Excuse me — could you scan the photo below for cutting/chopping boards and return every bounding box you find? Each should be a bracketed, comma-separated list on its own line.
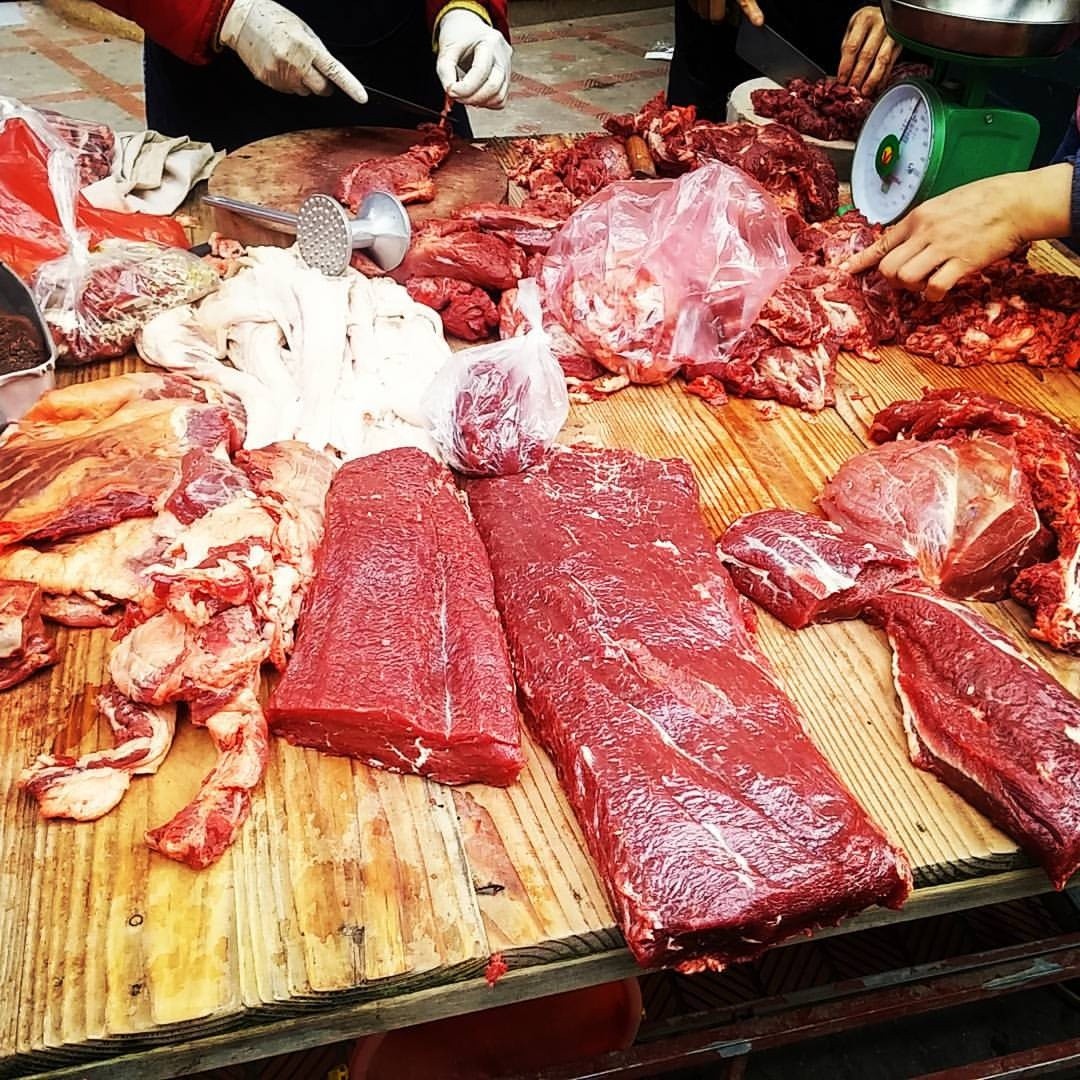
[728, 76, 857, 180]
[206, 128, 509, 248]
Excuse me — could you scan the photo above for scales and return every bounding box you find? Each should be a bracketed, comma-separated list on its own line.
[838, 1, 1079, 229]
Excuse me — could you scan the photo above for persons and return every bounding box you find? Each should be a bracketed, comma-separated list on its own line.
[835, 136, 1080, 303]
[664, 0, 905, 129]
[92, 0, 514, 145]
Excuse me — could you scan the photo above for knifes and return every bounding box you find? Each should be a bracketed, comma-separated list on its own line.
[724, 0, 825, 92]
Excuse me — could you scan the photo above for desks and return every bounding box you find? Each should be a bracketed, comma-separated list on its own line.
[0, 128, 1079, 1080]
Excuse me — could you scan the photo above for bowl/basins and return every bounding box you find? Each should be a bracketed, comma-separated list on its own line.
[0, 261, 60, 427]
